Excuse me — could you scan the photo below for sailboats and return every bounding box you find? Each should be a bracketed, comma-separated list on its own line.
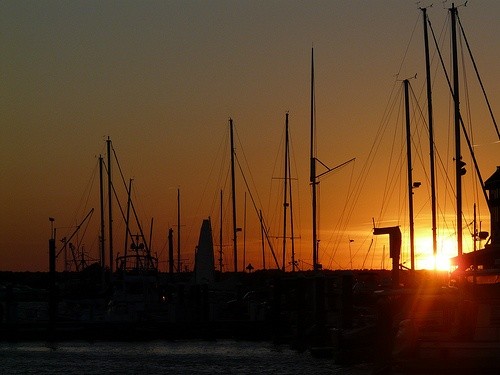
[8, 1, 497, 364]
